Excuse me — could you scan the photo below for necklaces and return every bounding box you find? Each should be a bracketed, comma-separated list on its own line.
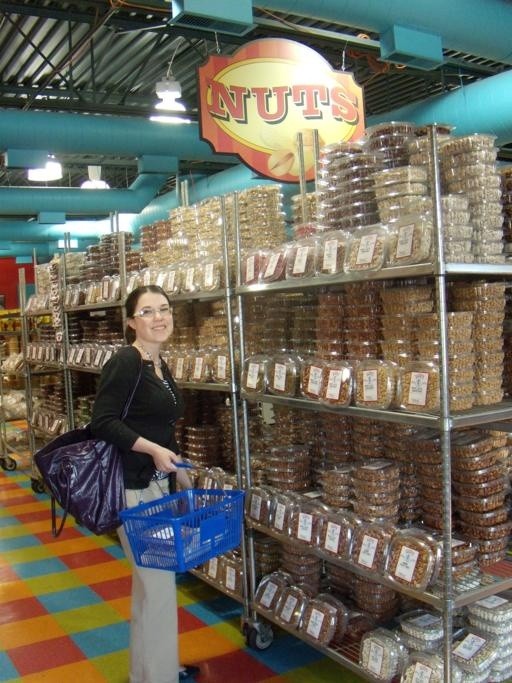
[134, 341, 163, 368]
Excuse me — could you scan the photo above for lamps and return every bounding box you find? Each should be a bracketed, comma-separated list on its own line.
[79, 177, 112, 189]
[148, 37, 191, 126]
[26, 156, 64, 183]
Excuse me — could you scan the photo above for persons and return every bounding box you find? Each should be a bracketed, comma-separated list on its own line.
[90, 281, 203, 682]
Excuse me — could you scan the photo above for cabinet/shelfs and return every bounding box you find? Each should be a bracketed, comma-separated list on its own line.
[124, 189, 250, 602]
[65, 223, 127, 536]
[2, 252, 63, 483]
[230, 130, 511, 641]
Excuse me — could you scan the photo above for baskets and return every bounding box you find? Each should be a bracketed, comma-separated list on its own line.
[117, 462, 245, 573]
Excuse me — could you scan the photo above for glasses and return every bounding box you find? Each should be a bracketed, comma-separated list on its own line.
[130, 306, 173, 320]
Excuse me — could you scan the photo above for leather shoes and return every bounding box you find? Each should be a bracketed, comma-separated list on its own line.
[178, 664, 201, 683]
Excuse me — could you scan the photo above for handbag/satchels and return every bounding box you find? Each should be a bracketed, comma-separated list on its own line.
[32, 424, 130, 539]
